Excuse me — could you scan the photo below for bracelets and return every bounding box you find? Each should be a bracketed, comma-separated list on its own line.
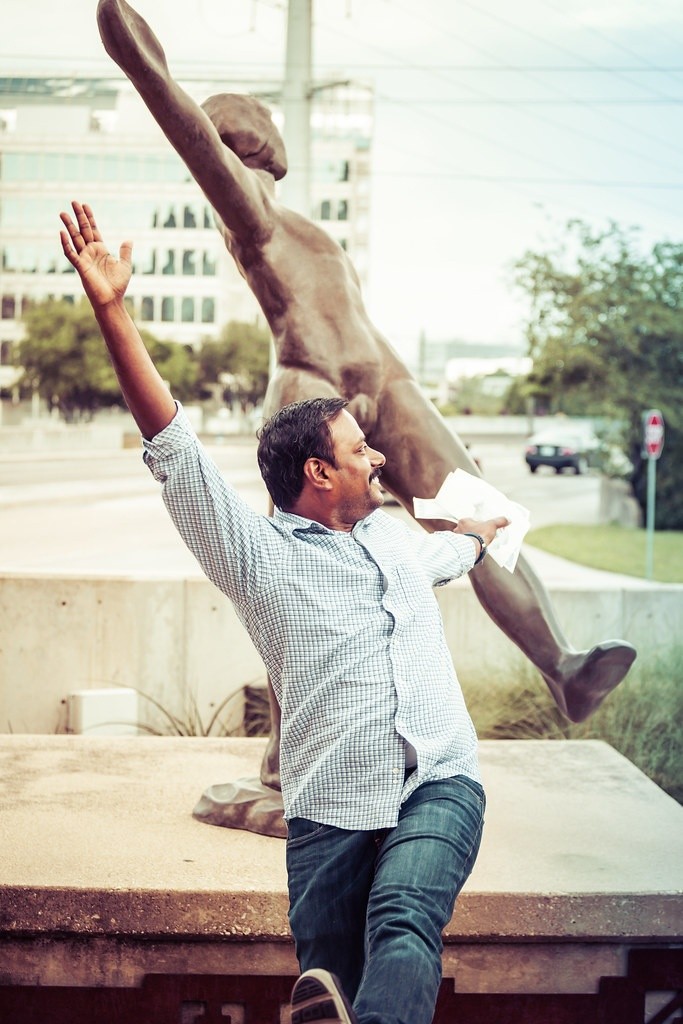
[464, 532, 486, 565]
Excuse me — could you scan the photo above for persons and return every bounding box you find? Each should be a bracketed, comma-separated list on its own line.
[96, 0, 638, 788]
[59, 201, 507, 1024]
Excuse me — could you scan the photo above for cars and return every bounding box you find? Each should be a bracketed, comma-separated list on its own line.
[525, 429, 610, 475]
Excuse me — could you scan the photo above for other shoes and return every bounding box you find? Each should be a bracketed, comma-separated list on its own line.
[288, 966, 361, 1024]
